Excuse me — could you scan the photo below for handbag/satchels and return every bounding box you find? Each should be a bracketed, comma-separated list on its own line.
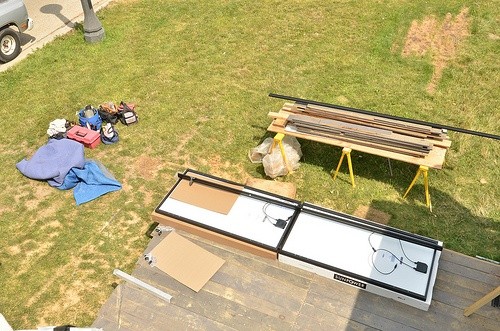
[98, 100, 138, 145]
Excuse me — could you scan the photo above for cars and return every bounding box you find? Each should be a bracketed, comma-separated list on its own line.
[0, 0, 33, 63]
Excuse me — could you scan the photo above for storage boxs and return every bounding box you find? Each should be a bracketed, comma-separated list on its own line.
[67, 124, 101, 149]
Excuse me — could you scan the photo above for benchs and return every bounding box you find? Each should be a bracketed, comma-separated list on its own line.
[333, 148, 355, 186]
[402, 165, 431, 209]
[266, 133, 290, 175]
